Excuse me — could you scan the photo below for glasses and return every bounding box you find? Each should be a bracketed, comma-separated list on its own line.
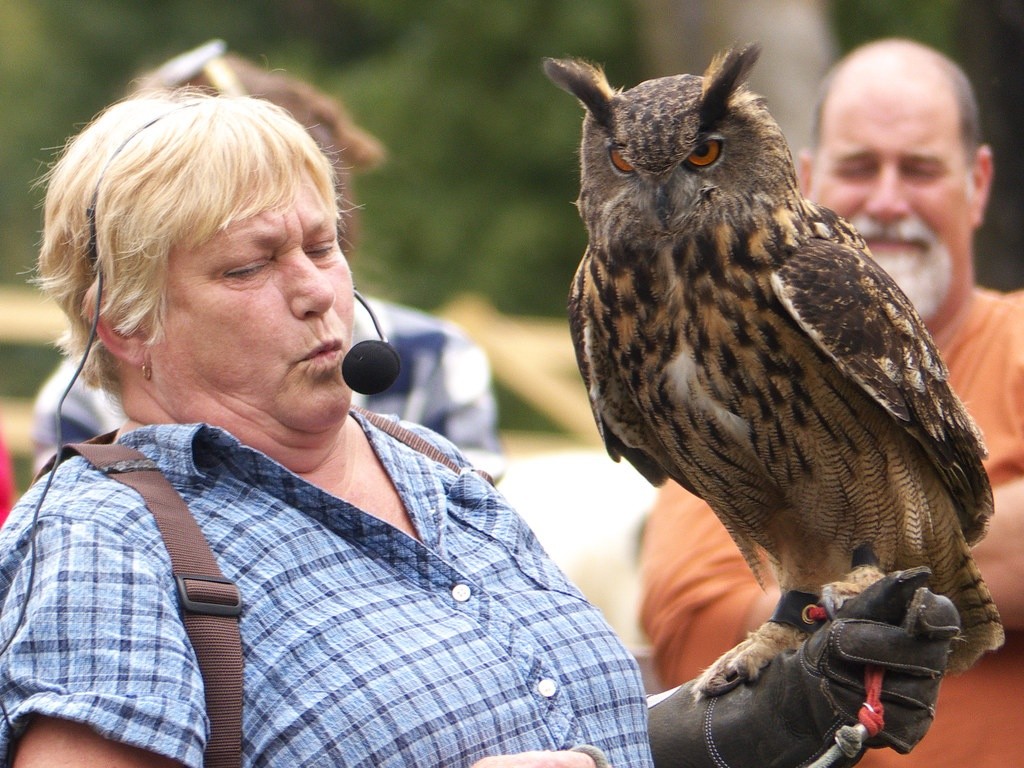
[154, 37, 246, 98]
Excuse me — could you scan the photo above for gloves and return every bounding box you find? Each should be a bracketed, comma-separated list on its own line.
[646, 543, 962, 768]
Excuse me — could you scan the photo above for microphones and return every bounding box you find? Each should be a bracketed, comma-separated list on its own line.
[342, 290, 401, 395]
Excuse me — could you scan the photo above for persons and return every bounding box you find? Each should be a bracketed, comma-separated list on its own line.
[634, 36, 1024, 768]
[29, 41, 504, 484]
[0, 88, 961, 768]
